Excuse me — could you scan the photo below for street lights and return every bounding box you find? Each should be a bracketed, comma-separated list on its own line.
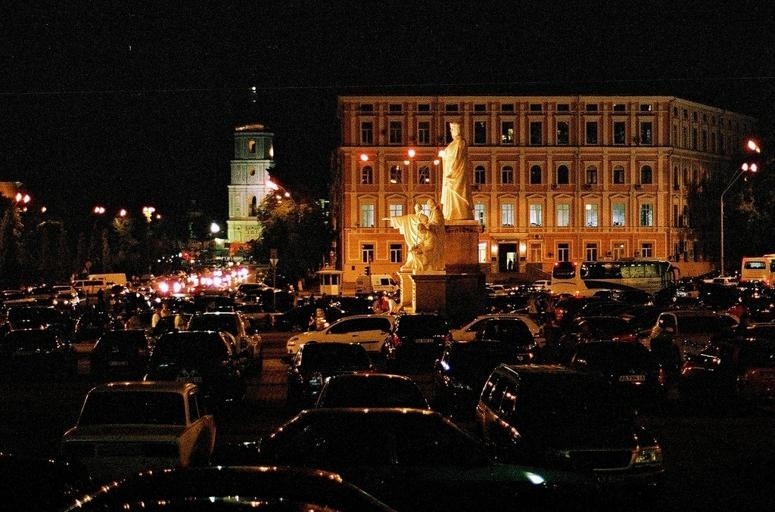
[360, 147, 441, 310]
[0, 192, 221, 254]
[721, 139, 762, 274]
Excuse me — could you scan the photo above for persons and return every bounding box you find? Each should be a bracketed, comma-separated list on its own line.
[433, 122, 475, 221]
[379, 198, 444, 272]
